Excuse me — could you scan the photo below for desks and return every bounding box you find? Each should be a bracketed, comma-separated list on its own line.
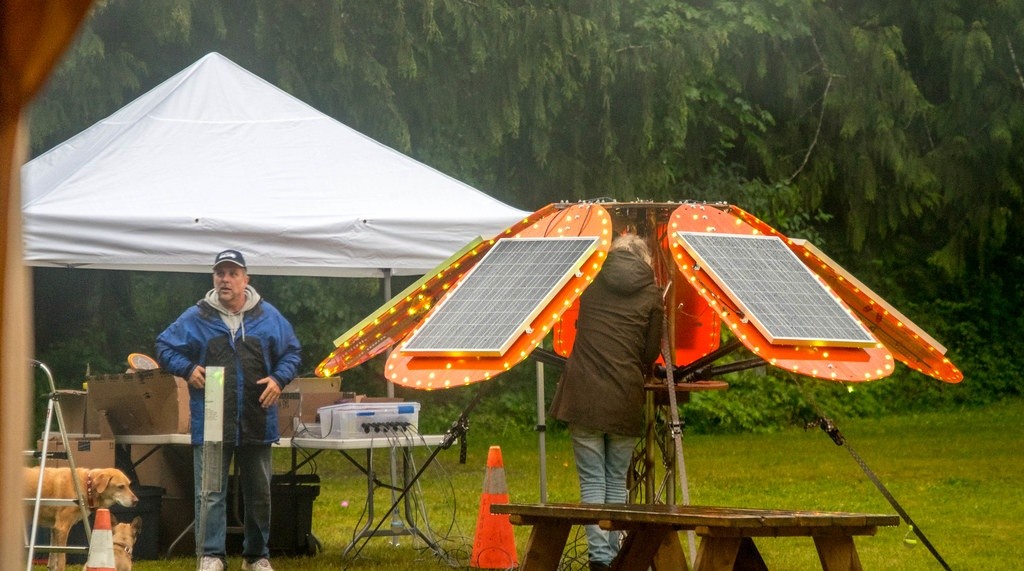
[490, 502, 900, 571]
[102, 432, 458, 561]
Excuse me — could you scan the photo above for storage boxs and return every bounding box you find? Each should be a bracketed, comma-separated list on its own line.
[37, 439, 115, 468]
[275, 376, 344, 437]
[56, 390, 114, 439]
[136, 368, 191, 434]
[317, 402, 421, 440]
[86, 373, 156, 434]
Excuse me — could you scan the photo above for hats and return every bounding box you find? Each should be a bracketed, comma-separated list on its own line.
[212, 250, 245, 270]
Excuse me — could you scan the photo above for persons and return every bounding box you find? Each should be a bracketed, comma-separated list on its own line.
[153, 250, 301, 570]
[553, 232, 665, 571]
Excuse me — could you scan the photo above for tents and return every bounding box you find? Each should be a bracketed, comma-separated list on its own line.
[19, 52, 546, 530]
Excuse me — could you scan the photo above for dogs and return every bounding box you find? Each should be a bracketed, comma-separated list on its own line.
[20, 466, 143, 570]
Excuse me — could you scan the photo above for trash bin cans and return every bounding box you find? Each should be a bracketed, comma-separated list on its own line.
[224, 474, 323, 557]
[109, 485, 166, 560]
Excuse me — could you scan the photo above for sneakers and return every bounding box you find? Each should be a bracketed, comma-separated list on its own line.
[242, 558, 273, 571]
[199, 556, 227, 571]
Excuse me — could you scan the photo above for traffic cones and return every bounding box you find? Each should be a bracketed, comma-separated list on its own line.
[465, 443, 520, 571]
[84, 507, 122, 571]
